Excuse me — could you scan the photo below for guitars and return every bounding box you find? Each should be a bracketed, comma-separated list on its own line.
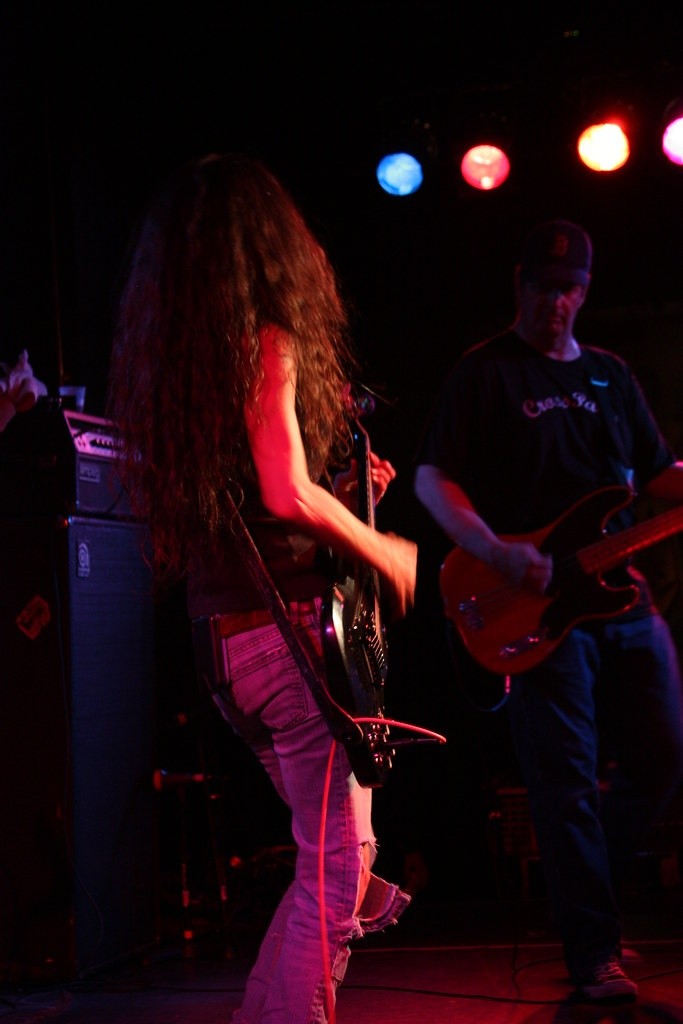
[321, 372, 412, 793]
[435, 480, 683, 676]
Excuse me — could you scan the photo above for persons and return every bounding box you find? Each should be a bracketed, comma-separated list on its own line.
[418, 223, 683, 1000]
[107, 150, 418, 1023]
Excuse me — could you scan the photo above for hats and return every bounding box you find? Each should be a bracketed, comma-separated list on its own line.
[516, 220, 592, 287]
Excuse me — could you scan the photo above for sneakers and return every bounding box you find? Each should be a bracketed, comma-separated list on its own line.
[571, 961, 640, 1004]
